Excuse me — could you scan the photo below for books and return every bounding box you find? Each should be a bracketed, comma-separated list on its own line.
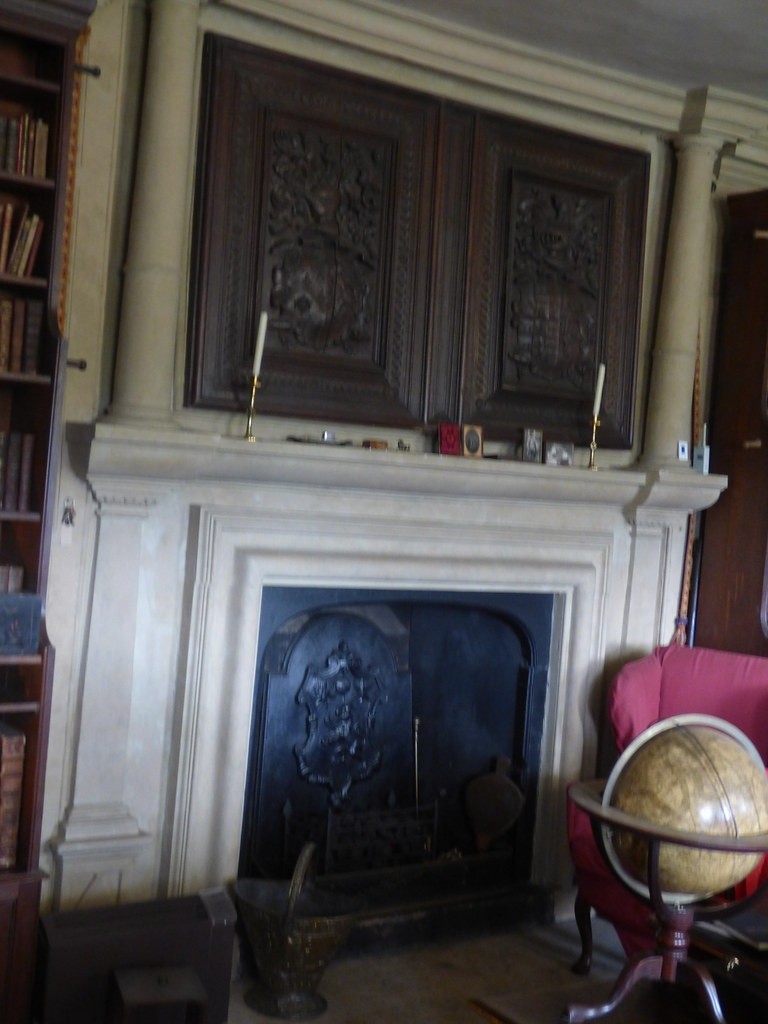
[0, 724, 25, 870]
[0, 107, 50, 593]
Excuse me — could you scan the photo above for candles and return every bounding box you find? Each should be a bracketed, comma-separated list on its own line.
[594, 364, 606, 415]
[252, 310, 269, 376]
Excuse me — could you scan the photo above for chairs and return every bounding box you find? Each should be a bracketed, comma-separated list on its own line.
[561, 645, 768, 1007]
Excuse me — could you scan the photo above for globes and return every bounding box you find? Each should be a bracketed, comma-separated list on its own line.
[562, 714, 768, 1024]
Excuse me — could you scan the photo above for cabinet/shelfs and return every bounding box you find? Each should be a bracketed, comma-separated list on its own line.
[0, 0, 103, 1022]
